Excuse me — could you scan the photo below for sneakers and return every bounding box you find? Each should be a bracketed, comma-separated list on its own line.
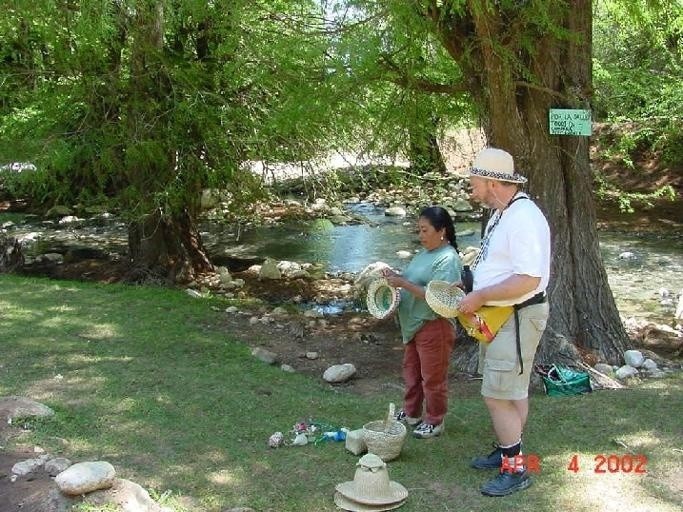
[481, 469, 531, 496]
[471, 442, 502, 468]
[412, 418, 445, 439]
[393, 409, 422, 425]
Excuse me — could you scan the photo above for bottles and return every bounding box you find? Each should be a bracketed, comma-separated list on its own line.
[461, 266, 472, 291]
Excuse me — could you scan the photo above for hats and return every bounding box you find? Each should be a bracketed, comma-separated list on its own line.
[459, 149, 529, 184]
[333, 453, 408, 512]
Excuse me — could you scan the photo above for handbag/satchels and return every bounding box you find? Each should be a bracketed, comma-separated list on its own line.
[543, 365, 592, 397]
[457, 307, 514, 344]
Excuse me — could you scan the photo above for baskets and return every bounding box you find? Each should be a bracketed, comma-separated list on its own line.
[362, 403, 407, 462]
[426, 281, 466, 318]
[366, 279, 401, 319]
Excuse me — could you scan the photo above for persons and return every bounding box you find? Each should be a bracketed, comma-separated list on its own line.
[450, 148, 551, 498]
[384, 207, 462, 439]
